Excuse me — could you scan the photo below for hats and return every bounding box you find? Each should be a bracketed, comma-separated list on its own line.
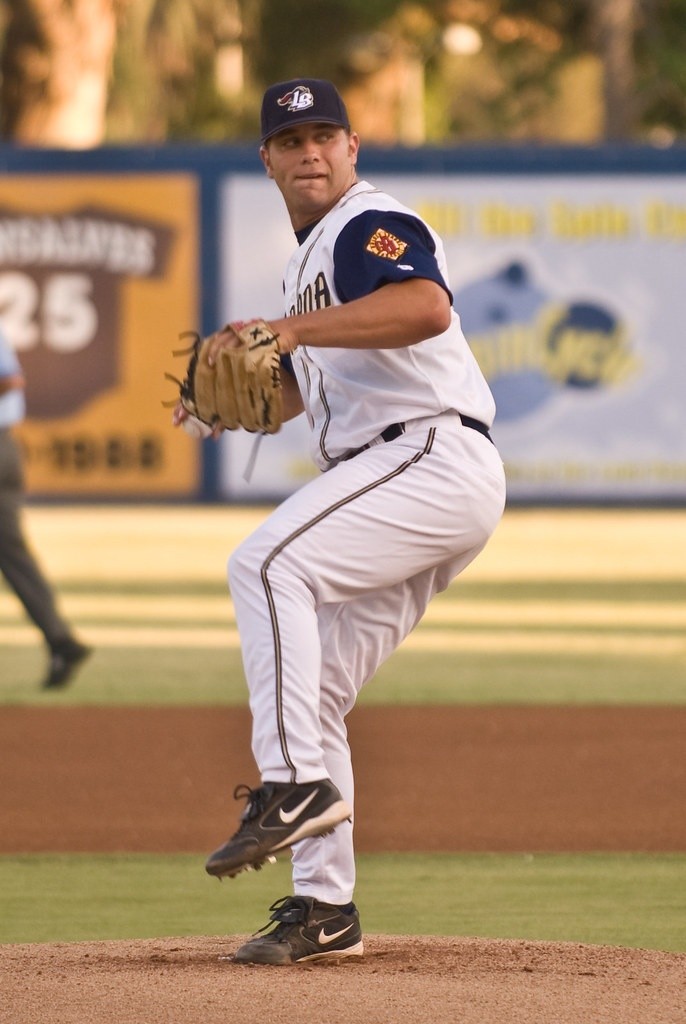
[257, 79, 350, 144]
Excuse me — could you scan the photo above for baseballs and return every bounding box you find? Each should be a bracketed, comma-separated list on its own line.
[182, 414, 213, 439]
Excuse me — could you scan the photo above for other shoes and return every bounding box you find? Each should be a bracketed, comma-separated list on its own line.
[39, 637, 89, 691]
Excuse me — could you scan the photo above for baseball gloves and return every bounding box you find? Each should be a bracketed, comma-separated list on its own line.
[180, 315, 285, 434]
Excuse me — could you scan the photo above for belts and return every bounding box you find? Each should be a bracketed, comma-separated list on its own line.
[352, 411, 491, 459]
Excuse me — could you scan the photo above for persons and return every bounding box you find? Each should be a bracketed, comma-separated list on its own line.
[174, 80, 507, 965]
[0, 331, 93, 689]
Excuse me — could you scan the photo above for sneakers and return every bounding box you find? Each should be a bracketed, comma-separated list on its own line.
[205, 778, 350, 880]
[231, 895, 364, 968]
